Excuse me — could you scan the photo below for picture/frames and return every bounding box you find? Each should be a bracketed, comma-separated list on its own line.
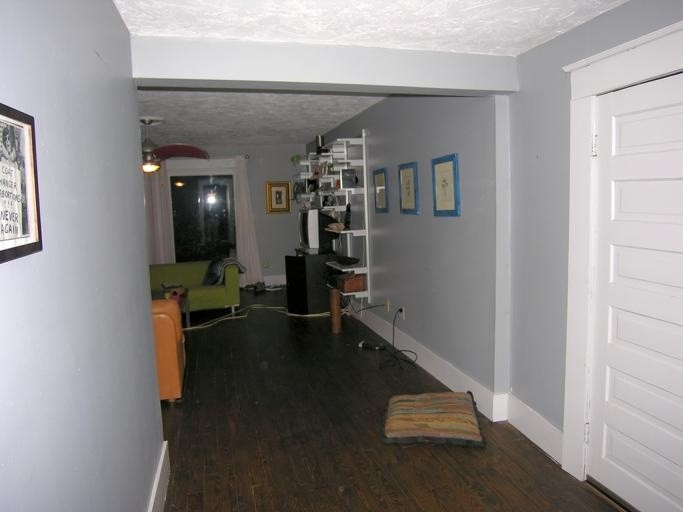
[0, 102, 42, 264]
[264, 179, 292, 216]
[372, 152, 463, 218]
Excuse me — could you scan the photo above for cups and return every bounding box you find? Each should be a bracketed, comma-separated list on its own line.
[315, 135, 324, 148]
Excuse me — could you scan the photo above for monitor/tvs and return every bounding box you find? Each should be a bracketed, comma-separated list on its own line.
[296, 208, 339, 250]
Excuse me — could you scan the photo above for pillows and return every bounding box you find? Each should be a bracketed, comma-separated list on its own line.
[380, 388, 487, 450]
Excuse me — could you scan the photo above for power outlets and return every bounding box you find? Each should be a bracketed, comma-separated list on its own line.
[383, 298, 389, 311]
[398, 305, 405, 320]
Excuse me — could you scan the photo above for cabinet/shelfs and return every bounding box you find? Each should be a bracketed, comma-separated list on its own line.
[299, 128, 370, 303]
[284, 248, 337, 315]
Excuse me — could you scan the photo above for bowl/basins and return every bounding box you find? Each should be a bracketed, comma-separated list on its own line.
[332, 255, 359, 266]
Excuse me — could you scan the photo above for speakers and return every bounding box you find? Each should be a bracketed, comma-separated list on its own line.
[305, 178, 315, 192]
[339, 168, 355, 189]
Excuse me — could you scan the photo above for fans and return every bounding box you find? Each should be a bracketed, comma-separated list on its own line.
[138, 118, 210, 162]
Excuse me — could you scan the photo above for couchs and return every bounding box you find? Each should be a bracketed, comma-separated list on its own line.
[151, 299, 186, 403]
[149, 259, 241, 316]
